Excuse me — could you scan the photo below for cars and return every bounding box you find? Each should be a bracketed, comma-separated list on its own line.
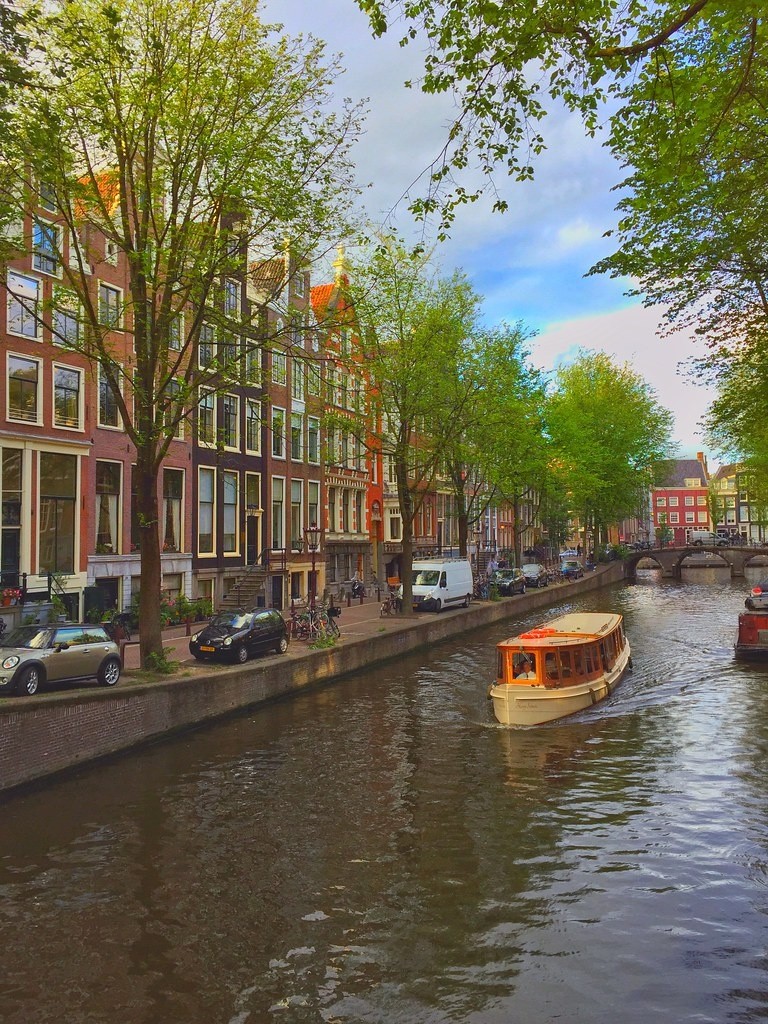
[559, 549, 577, 559]
[520, 563, 549, 588]
[559, 560, 584, 579]
[188, 605, 290, 665]
[0, 621, 122, 696]
[496, 567, 526, 598]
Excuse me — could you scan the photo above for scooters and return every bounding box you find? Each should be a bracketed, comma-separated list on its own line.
[350, 579, 365, 599]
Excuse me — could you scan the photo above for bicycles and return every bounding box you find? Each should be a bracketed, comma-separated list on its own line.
[286, 596, 341, 643]
[380, 591, 399, 616]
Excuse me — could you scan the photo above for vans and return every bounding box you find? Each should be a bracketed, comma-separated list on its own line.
[397, 555, 474, 614]
[690, 531, 730, 547]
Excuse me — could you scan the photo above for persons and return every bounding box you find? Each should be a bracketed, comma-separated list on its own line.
[723, 530, 764, 548]
[543, 644, 599, 679]
[577, 543, 582, 557]
[633, 537, 675, 550]
[513, 659, 536, 680]
[625, 539, 629, 544]
[489, 555, 509, 569]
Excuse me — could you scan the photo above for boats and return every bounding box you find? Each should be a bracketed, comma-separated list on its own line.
[732, 574, 768, 655]
[489, 612, 632, 728]
